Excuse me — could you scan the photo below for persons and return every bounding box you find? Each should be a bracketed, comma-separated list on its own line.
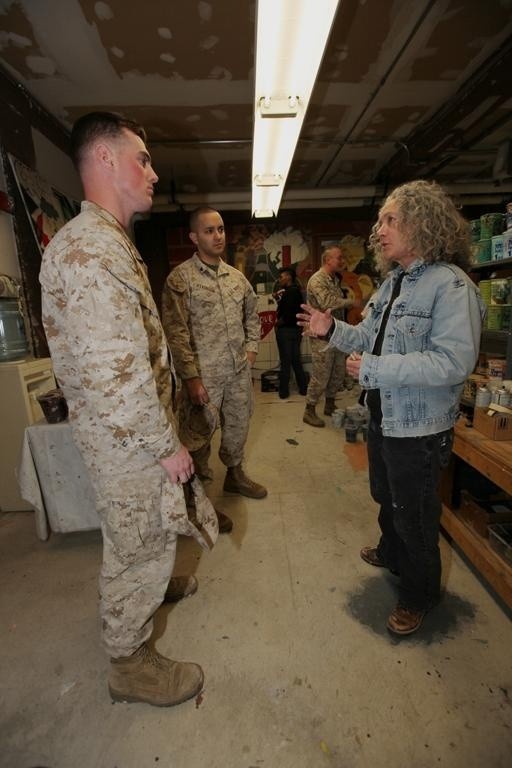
[39, 110, 219, 706]
[302, 246, 355, 427]
[296, 180, 488, 636]
[160, 206, 268, 534]
[272, 267, 307, 399]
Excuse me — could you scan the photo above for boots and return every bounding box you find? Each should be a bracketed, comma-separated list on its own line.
[164, 575, 198, 602]
[108, 642, 204, 707]
[303, 404, 325, 427]
[324, 397, 339, 416]
[223, 464, 267, 499]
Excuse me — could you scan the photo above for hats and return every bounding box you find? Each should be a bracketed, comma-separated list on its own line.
[160, 474, 219, 551]
[179, 401, 218, 453]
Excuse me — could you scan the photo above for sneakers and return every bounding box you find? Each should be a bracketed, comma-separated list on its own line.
[360, 546, 384, 567]
[386, 600, 426, 634]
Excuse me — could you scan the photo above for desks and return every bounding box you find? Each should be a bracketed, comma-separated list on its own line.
[435, 406, 512, 614]
[20, 417, 99, 540]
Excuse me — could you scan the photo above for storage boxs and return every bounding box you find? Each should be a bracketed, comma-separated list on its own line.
[474, 402, 512, 440]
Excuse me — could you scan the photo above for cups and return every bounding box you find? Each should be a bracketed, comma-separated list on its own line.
[331, 405, 370, 444]
[35, 393, 65, 425]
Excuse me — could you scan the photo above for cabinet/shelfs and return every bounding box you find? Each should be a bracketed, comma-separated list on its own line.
[463, 255, 512, 431]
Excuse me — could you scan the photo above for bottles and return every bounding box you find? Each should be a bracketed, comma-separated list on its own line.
[494, 385, 512, 407]
[475, 384, 492, 408]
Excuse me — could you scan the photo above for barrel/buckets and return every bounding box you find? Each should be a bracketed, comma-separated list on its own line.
[0, 296, 29, 362]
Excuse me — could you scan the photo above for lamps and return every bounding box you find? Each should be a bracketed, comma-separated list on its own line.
[251, 0, 341, 218]
